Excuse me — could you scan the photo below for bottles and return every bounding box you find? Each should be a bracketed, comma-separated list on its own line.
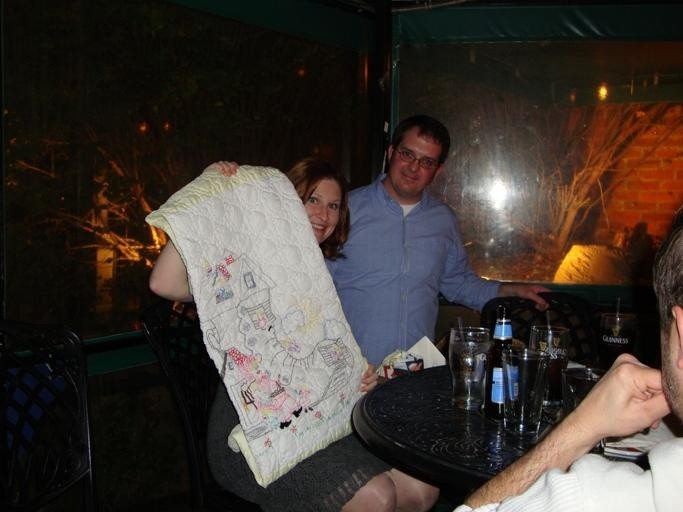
[490, 305, 521, 414]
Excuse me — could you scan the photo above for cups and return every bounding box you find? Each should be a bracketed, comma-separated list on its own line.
[447, 324, 489, 411]
[502, 346, 546, 438]
[600, 313, 637, 360]
[562, 366, 601, 410]
[530, 324, 569, 408]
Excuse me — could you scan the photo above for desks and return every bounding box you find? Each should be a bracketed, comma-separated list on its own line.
[352, 358, 650, 495]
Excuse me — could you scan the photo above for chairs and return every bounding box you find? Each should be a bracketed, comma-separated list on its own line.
[140, 300, 261, 510]
[480, 292, 608, 369]
[0, 317, 96, 512]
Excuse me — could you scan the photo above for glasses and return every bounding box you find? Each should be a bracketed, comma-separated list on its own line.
[395, 148, 436, 169]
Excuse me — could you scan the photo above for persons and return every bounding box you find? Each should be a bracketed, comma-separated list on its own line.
[458, 225, 681, 508]
[319, 115, 553, 376]
[149, 157, 441, 508]
